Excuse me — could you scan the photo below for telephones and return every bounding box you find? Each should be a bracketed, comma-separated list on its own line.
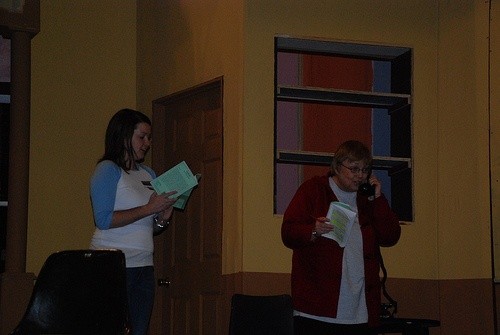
[359, 172, 375, 197]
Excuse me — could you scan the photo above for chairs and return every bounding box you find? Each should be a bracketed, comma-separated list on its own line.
[9, 250, 127, 335]
[229, 294, 293, 335]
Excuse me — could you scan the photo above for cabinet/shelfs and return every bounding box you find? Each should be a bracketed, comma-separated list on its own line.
[274, 37, 413, 224]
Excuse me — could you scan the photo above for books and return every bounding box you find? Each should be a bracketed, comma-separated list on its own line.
[149, 161, 204, 209]
[321, 203, 358, 247]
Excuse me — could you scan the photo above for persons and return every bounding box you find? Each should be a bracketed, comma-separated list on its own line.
[282, 140, 401, 334]
[89, 108, 178, 335]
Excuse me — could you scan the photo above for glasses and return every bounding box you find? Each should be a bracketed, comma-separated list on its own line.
[340, 162, 371, 174]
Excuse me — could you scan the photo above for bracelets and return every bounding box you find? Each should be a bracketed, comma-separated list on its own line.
[155, 218, 170, 228]
